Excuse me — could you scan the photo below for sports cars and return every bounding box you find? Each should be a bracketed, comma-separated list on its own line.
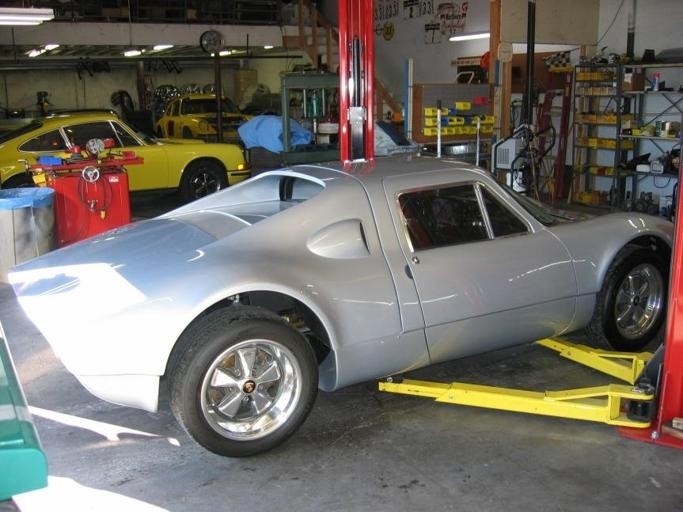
[0, 111, 245, 204]
[7, 154, 673, 457]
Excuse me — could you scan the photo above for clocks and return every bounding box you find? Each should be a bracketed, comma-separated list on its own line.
[496, 42, 512, 63]
[200, 29, 225, 56]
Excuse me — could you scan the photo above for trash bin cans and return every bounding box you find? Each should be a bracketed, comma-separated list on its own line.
[0, 187, 56, 283]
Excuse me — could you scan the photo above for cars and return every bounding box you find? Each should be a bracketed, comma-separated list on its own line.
[154, 96, 254, 146]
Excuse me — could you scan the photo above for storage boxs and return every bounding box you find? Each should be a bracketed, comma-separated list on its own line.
[621, 72, 643, 93]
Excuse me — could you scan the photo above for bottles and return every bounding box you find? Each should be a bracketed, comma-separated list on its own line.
[652, 72, 660, 91]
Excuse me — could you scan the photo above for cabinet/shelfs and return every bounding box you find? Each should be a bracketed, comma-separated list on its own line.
[620, 59, 683, 222]
[568, 59, 626, 209]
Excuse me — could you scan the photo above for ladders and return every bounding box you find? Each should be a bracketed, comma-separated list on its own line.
[534, 65, 574, 204]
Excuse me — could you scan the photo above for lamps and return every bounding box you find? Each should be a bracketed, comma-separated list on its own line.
[0, 0, 55, 27]
[447, 32, 490, 42]
